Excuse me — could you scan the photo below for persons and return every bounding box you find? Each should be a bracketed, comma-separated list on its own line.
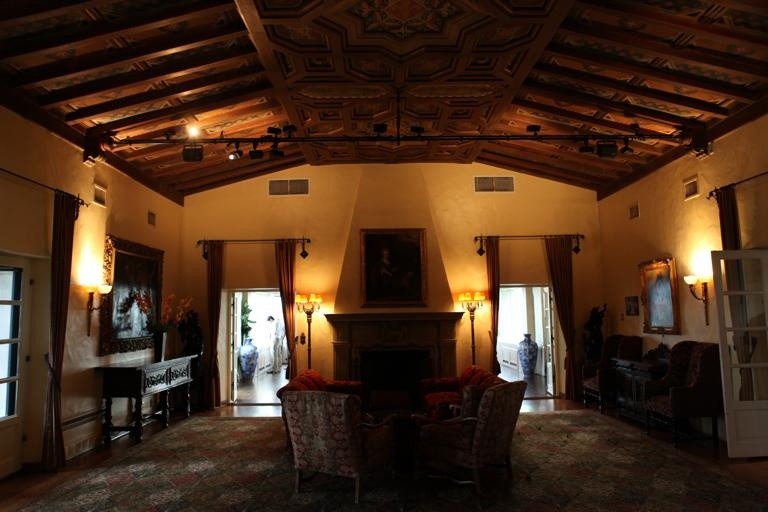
[266, 314, 286, 374]
[378, 248, 394, 278]
[650, 272, 672, 316]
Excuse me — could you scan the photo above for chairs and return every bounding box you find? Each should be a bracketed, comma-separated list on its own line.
[426, 381, 529, 511]
[282, 391, 394, 505]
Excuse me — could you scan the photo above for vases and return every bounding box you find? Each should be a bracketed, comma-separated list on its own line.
[518, 334, 538, 380]
[238, 339, 258, 384]
[152, 331, 167, 362]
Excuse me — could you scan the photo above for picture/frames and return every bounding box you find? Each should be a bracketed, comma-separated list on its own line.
[623, 296, 639, 316]
[637, 257, 679, 335]
[99, 235, 164, 356]
[359, 227, 429, 307]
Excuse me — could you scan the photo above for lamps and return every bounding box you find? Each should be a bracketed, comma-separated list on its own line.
[683, 250, 716, 326]
[294, 293, 323, 370]
[99, 87, 693, 161]
[459, 292, 483, 366]
[87, 237, 115, 336]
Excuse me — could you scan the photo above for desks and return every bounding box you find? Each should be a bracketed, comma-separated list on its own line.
[612, 342, 671, 423]
[93, 353, 199, 444]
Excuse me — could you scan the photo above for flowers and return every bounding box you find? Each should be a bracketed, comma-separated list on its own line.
[135, 290, 194, 331]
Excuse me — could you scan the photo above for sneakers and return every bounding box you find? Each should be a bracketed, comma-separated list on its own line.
[267, 371, 276, 374]
[277, 369, 280, 373]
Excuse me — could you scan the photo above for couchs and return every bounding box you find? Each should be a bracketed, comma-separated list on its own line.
[579, 334, 643, 415]
[639, 341, 723, 444]
[411, 367, 508, 463]
[277, 370, 363, 447]
